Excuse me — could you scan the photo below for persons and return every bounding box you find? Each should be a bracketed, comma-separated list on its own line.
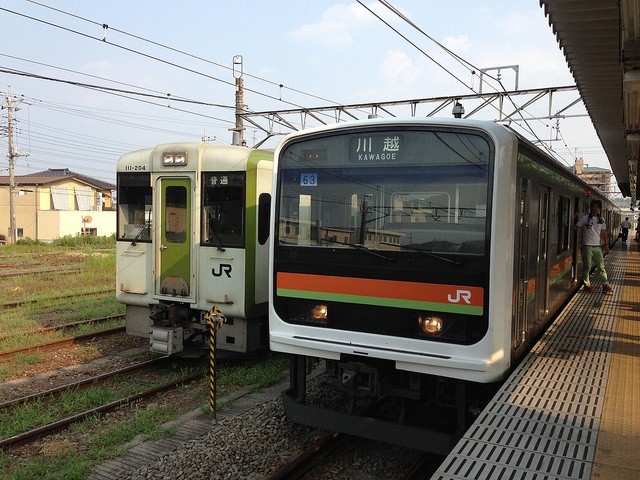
[621, 217, 631, 241]
[634, 219, 640, 240]
[575, 200, 614, 293]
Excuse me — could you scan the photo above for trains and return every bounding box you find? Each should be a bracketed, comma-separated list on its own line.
[269, 116, 622, 457]
[115, 142, 274, 356]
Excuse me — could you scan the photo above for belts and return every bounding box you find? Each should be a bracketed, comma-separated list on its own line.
[584, 245, 600, 247]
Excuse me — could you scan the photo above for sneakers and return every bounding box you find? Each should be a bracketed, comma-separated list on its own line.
[585, 286, 595, 291]
[603, 285, 614, 292]
